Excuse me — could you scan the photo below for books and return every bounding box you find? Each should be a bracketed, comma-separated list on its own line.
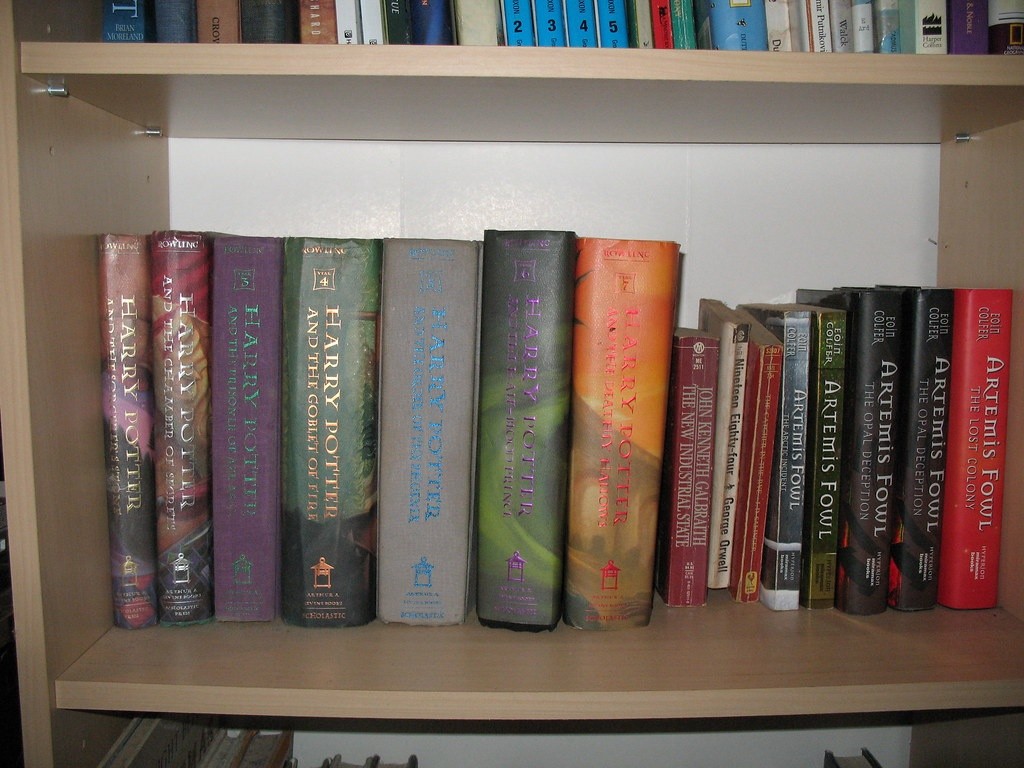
[101, 0, 1024, 54]
[824, 747, 882, 768]
[96, 229, 1014, 640]
[96, 718, 418, 768]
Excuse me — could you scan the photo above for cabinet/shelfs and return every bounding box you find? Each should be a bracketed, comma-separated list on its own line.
[0, 0, 1024, 768]
